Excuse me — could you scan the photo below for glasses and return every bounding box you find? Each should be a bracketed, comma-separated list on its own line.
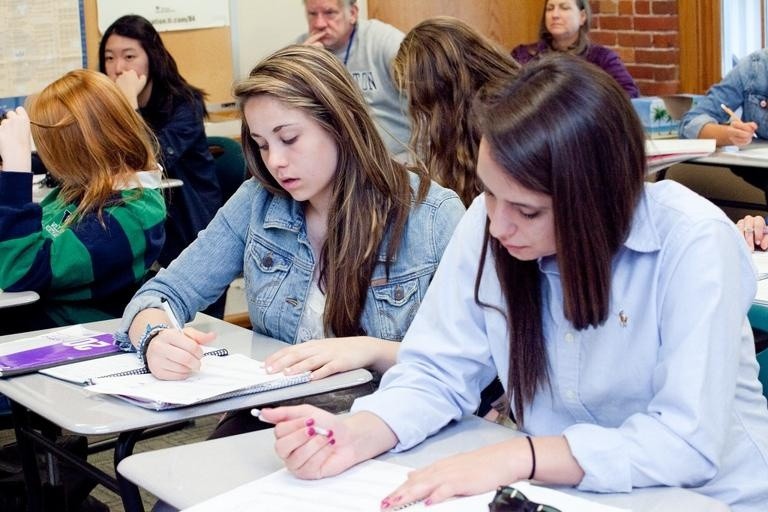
[488, 485, 560, 511]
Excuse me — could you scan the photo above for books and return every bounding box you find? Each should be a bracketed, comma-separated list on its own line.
[42, 343, 312, 413]
[643, 137, 719, 175]
[178, 456, 499, 512]
[720, 147, 767, 160]
[0, 332, 123, 378]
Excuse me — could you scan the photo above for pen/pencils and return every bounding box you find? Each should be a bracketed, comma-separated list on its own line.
[161, 296, 187, 338]
[249, 407, 331, 439]
[720, 103, 759, 140]
[742, 226, 768, 235]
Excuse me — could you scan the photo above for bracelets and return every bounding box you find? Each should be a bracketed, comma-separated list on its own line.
[135, 323, 170, 374]
[524, 435, 538, 479]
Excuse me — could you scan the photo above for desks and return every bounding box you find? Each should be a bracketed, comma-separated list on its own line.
[660, 132, 768, 217]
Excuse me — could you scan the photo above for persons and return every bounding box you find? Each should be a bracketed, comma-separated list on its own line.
[294, 0, 415, 170]
[735, 212, 768, 253]
[97, 13, 225, 276]
[675, 47, 768, 149]
[256, 51, 768, 512]
[506, 1, 641, 99]
[109, 44, 470, 512]
[0, 67, 169, 475]
